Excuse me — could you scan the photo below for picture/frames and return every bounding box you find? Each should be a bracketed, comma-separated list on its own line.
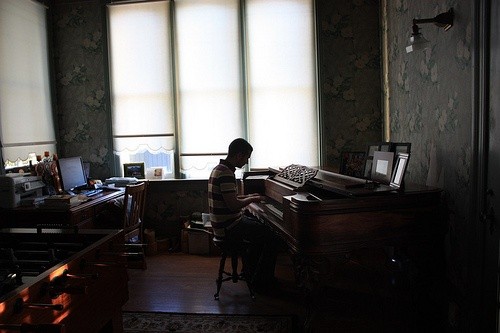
[365, 142, 411, 189]
[124, 163, 145, 179]
[338, 151, 365, 176]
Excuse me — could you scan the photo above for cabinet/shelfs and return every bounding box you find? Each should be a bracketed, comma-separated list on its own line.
[0, 184, 124, 229]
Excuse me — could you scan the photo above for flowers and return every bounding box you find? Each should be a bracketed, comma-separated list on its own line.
[35, 152, 60, 186]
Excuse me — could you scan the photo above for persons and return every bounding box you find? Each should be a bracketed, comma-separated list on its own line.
[207, 138, 279, 295]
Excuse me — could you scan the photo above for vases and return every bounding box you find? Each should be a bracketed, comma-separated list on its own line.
[48, 183, 60, 196]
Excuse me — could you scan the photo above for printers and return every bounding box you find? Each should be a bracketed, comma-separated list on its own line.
[0, 173, 46, 209]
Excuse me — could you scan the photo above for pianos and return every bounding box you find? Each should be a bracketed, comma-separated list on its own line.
[243, 166, 443, 292]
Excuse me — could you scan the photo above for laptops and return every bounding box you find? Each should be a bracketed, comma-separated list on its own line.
[56, 156, 103, 196]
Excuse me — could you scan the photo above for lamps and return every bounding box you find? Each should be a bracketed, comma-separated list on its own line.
[405, 7, 454, 54]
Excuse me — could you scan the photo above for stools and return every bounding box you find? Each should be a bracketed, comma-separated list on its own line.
[213, 238, 261, 301]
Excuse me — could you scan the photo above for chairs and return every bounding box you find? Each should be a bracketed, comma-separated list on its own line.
[120, 181, 148, 269]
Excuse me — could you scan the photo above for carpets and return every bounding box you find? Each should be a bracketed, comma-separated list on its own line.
[122, 310, 304, 333]
